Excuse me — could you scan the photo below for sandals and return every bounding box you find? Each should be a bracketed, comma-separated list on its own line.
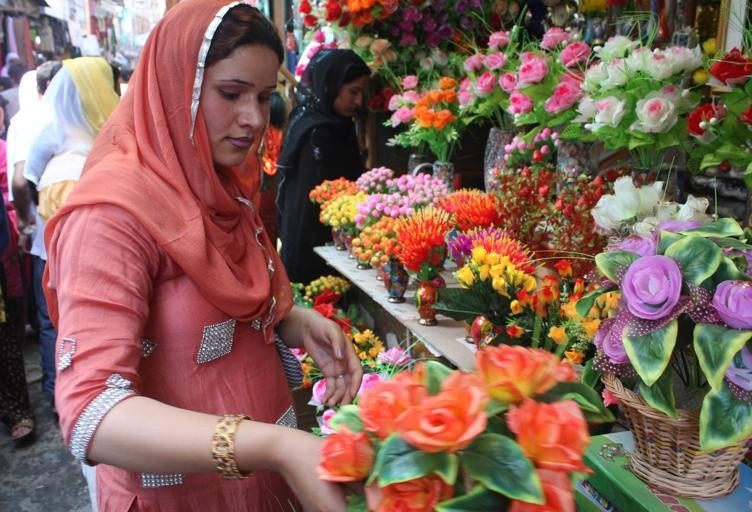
[11, 417, 35, 441]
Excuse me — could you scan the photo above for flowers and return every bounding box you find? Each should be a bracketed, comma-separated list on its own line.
[260, 0, 752, 512]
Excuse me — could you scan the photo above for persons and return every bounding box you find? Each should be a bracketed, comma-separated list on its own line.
[1, 51, 134, 448]
[48, 1, 370, 511]
[263, 48, 371, 290]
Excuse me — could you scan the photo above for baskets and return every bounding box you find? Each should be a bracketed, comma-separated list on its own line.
[600, 366, 752, 499]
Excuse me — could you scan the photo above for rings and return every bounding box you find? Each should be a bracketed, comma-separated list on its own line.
[336, 374, 344, 378]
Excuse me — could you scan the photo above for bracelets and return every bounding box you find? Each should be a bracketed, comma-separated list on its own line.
[213, 414, 254, 481]
